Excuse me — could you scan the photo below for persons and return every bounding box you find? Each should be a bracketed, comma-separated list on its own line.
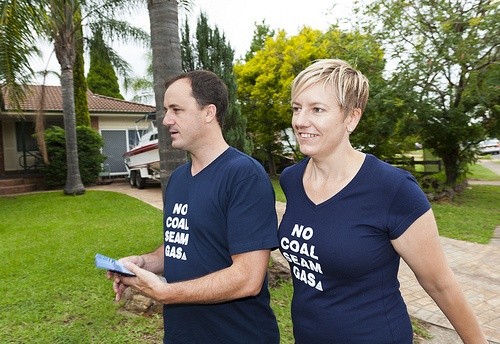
[277, 59, 489, 344]
[107, 70, 280, 344]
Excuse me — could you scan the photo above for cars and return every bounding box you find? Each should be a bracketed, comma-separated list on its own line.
[479, 140, 500, 155]
